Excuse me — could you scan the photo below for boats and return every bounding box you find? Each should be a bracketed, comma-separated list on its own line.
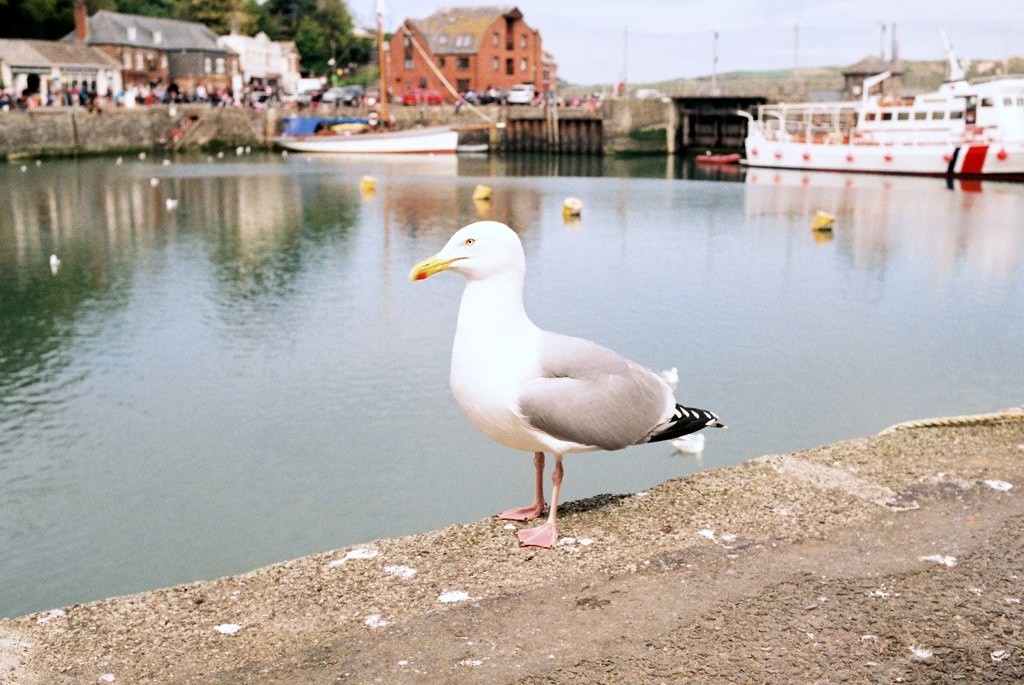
[737, 24, 1024, 177]
[696, 153, 740, 162]
[269, 122, 490, 154]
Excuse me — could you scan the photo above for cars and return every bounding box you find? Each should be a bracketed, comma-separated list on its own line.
[479, 83, 537, 105]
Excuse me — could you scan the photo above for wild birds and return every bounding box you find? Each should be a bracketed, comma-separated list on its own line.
[410, 218, 731, 548]
[20, 143, 313, 212]
[47, 252, 61, 269]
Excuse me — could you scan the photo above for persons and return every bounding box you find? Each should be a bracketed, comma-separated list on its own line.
[454, 87, 508, 113]
[171, 113, 199, 144]
[532, 90, 603, 109]
[0, 77, 394, 115]
